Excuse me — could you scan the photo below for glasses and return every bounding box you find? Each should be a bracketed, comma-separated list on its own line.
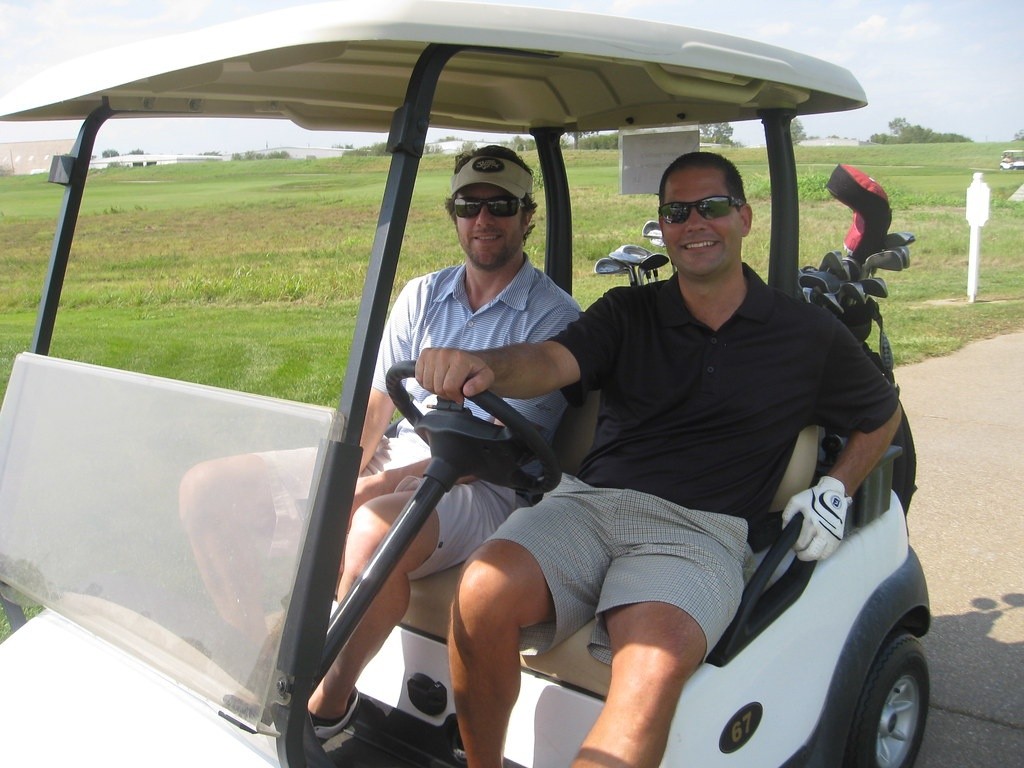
[454, 194, 520, 218]
[657, 195, 745, 224]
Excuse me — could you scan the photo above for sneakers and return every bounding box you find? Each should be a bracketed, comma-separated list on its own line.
[307, 684, 360, 745]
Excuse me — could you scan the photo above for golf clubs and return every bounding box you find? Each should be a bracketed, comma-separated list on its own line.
[793, 158, 917, 314]
[591, 220, 676, 287]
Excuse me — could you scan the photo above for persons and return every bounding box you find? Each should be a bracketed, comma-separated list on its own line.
[413, 151, 904, 768]
[177, 144, 580, 767]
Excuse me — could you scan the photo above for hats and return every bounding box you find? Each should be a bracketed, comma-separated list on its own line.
[450, 155, 533, 199]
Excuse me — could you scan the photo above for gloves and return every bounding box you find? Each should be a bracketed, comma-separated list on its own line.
[781, 475, 853, 562]
